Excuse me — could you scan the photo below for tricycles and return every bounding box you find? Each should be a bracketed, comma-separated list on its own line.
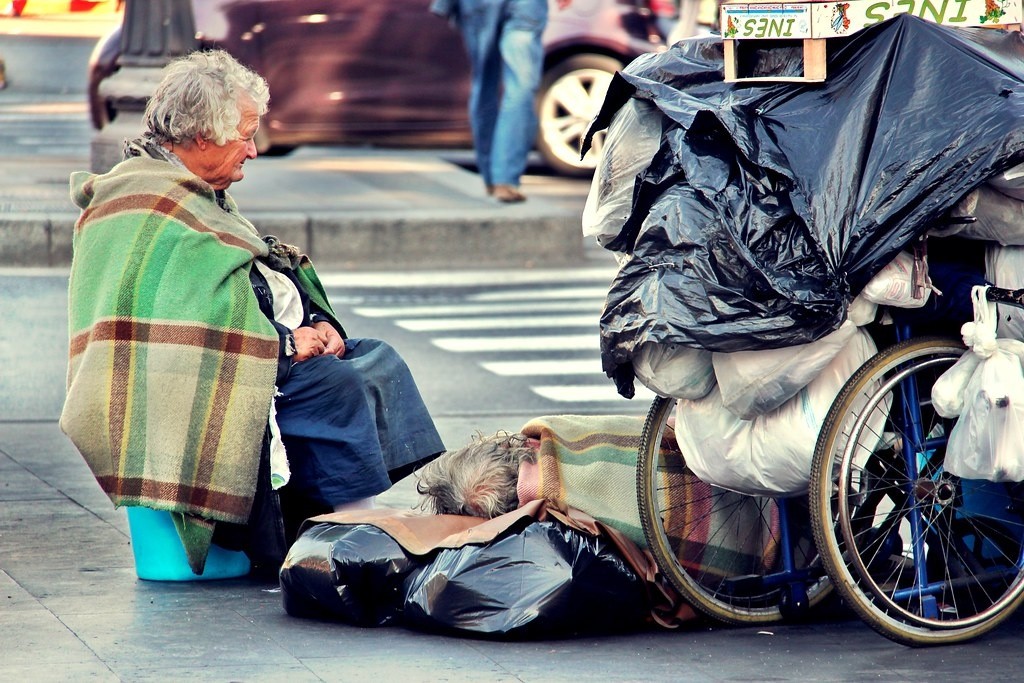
[633, 208, 1024, 648]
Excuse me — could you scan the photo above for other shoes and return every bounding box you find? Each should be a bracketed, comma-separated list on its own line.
[487, 186, 524, 202]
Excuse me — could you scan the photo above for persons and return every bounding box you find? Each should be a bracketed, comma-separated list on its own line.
[61, 53, 446, 574]
[431, 0, 570, 204]
[415, 414, 904, 632]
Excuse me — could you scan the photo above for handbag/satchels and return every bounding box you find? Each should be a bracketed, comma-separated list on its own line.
[932, 286, 984, 420]
[943, 285, 1024, 483]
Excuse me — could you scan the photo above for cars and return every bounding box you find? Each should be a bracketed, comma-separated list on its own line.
[85, 0, 675, 181]
